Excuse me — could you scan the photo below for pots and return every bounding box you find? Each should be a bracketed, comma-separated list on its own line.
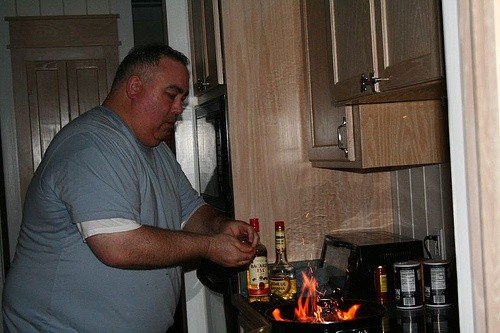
[263, 292, 384, 333]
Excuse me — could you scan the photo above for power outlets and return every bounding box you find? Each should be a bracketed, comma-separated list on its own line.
[433, 227, 446, 261]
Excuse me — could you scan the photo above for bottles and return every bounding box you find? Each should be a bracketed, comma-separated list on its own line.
[247, 218, 270, 303]
[375, 265, 388, 304]
[270, 221, 297, 303]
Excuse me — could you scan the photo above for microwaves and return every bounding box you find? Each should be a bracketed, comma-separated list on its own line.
[319, 231, 422, 280]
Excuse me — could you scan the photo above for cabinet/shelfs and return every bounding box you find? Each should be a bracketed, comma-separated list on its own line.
[187, 0, 447, 264]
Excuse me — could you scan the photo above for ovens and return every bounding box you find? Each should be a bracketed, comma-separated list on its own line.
[192, 94, 241, 295]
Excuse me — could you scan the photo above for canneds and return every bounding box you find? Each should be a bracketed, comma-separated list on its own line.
[393, 261, 423, 310]
[423, 260, 453, 308]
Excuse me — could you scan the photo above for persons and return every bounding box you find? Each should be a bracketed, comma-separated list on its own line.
[0, 45, 260, 333]
